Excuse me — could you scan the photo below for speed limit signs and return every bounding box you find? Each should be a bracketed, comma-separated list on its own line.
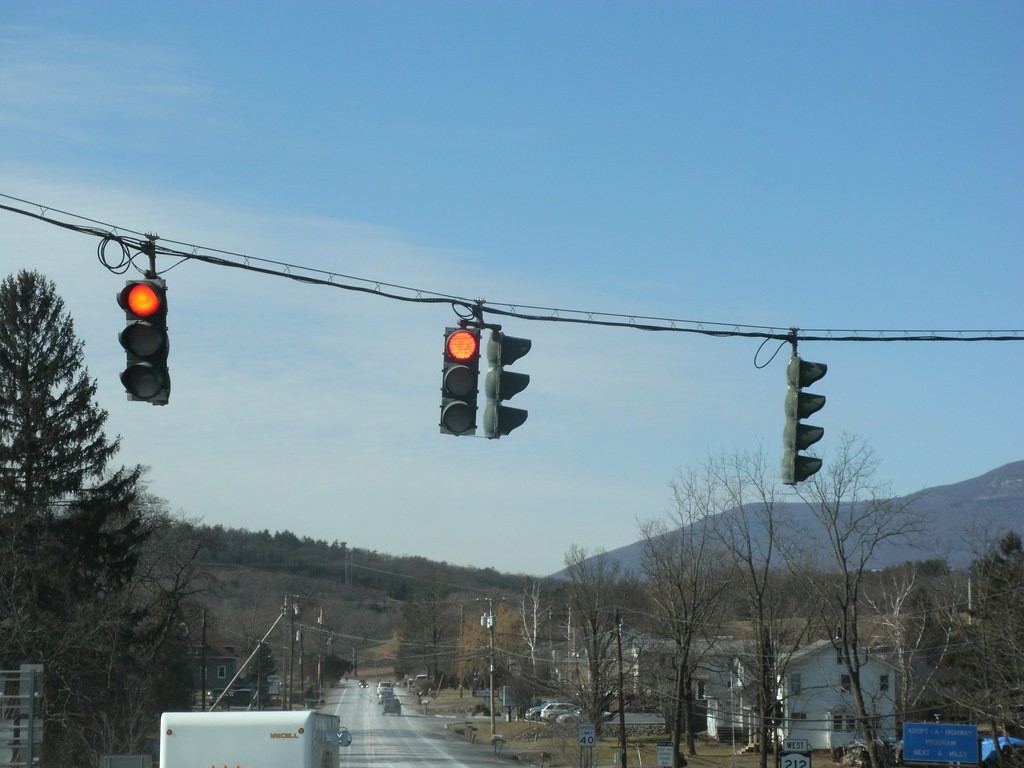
[579, 724, 595, 747]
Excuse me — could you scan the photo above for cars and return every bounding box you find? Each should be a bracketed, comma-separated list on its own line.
[376, 682, 394, 695]
[382, 698, 401, 716]
[556, 708, 613, 725]
[232, 668, 314, 711]
[207, 688, 228, 707]
[524, 701, 568, 721]
[376, 690, 395, 705]
[359, 680, 369, 688]
[540, 703, 580, 722]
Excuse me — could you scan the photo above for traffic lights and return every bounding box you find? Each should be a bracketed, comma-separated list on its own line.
[117, 274, 171, 404]
[780, 356, 825, 485]
[442, 322, 530, 442]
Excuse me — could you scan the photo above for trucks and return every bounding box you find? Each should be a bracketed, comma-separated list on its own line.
[159, 708, 352, 768]
